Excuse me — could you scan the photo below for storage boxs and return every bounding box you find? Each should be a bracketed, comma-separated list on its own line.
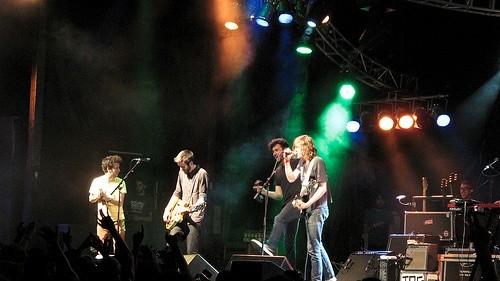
[403, 211, 456, 241]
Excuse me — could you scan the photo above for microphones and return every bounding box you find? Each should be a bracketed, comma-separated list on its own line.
[483, 158, 498, 170]
[133, 157, 150, 162]
[281, 151, 296, 156]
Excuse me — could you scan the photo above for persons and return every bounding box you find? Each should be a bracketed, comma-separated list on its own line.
[283, 135, 337, 281]
[89, 155, 127, 255]
[250, 138, 306, 269]
[163, 151, 208, 279]
[126, 178, 155, 248]
[449, 181, 491, 249]
[368, 192, 393, 251]
[0, 210, 193, 281]
[465, 213, 500, 281]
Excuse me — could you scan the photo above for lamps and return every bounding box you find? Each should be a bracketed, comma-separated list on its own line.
[296, 30, 313, 54]
[223, 0, 244, 30]
[378, 110, 415, 132]
[276, 0, 295, 24]
[255, 0, 275, 27]
[306, 0, 329, 28]
[429, 105, 451, 127]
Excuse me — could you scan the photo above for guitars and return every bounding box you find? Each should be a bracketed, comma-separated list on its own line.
[421, 176, 428, 212]
[440, 178, 448, 211]
[449, 173, 458, 195]
[293, 194, 308, 219]
[165, 199, 206, 230]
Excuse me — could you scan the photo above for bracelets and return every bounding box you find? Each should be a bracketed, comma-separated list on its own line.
[373, 223, 376, 229]
[283, 158, 290, 162]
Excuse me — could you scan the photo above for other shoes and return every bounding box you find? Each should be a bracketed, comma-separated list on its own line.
[251, 239, 275, 256]
[325, 277, 337, 281]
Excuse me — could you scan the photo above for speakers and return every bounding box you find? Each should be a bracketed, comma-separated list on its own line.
[224, 259, 286, 281]
[442, 259, 500, 281]
[386, 234, 424, 256]
[177, 253, 219, 281]
[333, 250, 393, 281]
[226, 254, 293, 271]
[404, 211, 453, 241]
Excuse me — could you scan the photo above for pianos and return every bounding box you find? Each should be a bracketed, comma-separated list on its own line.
[447, 199, 500, 281]
